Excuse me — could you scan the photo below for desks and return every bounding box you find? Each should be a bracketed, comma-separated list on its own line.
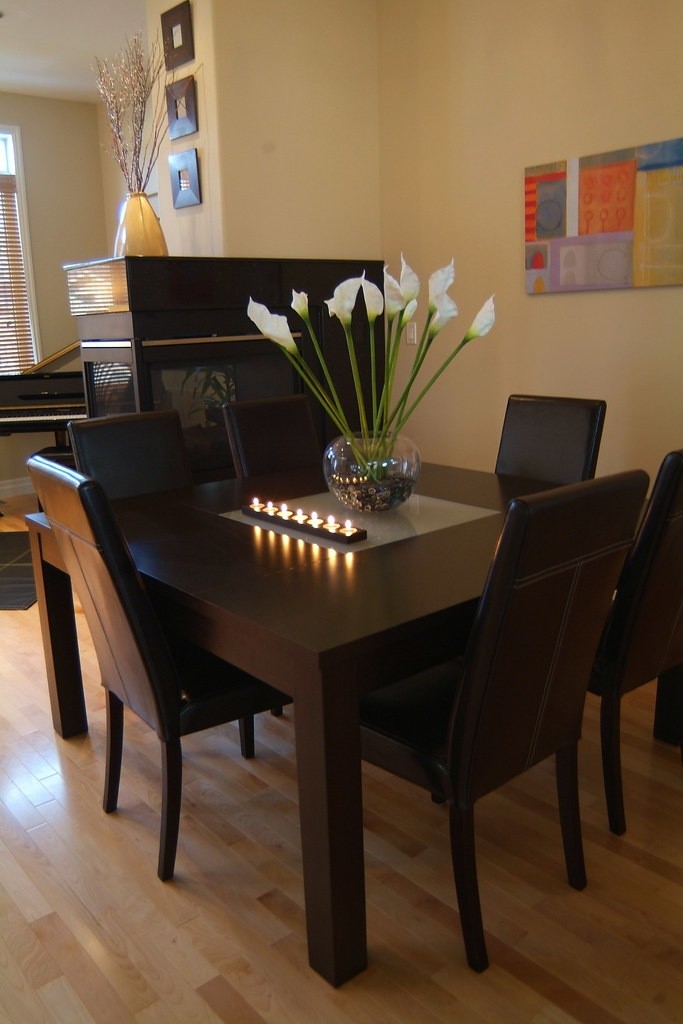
[26, 462, 574, 988]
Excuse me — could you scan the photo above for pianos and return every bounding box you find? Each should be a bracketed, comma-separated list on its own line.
[0, 369, 89, 514]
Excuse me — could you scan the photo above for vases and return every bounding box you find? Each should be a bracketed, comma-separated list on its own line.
[114, 192, 169, 257]
[323, 432, 421, 511]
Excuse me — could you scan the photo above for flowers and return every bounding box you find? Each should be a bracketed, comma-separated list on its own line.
[247, 251, 494, 483]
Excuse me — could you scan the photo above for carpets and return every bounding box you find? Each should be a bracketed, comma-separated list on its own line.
[0, 531, 37, 609]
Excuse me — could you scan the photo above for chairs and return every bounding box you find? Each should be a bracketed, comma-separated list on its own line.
[360, 468, 650, 973]
[495, 394, 608, 485]
[586, 449, 683, 835]
[222, 395, 321, 479]
[67, 408, 282, 717]
[25, 454, 292, 880]
